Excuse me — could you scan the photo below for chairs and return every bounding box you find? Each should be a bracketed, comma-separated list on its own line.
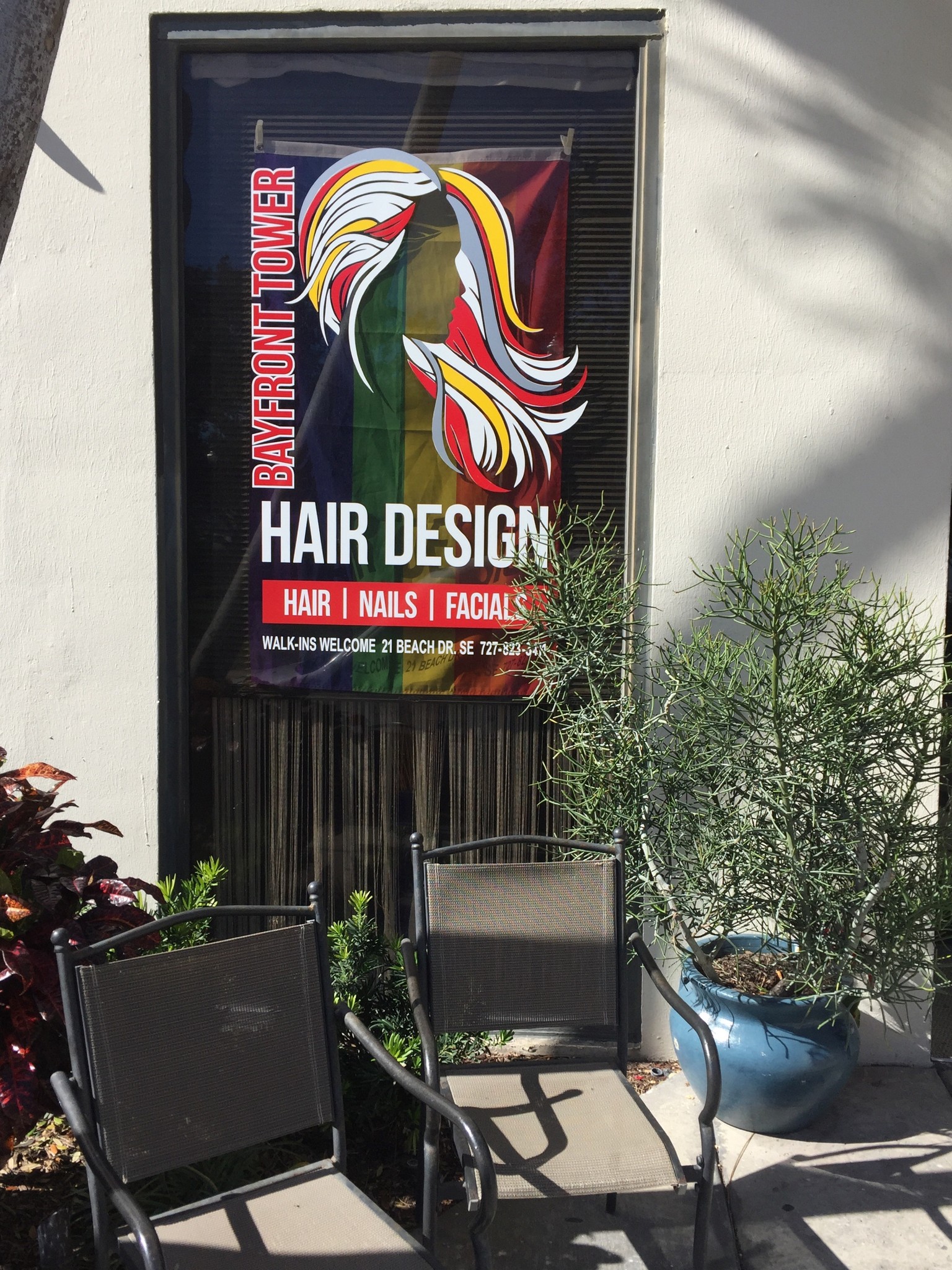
[50, 829, 721, 1270]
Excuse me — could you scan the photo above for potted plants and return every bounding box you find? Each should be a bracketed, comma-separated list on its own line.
[502, 489, 952, 1133]
